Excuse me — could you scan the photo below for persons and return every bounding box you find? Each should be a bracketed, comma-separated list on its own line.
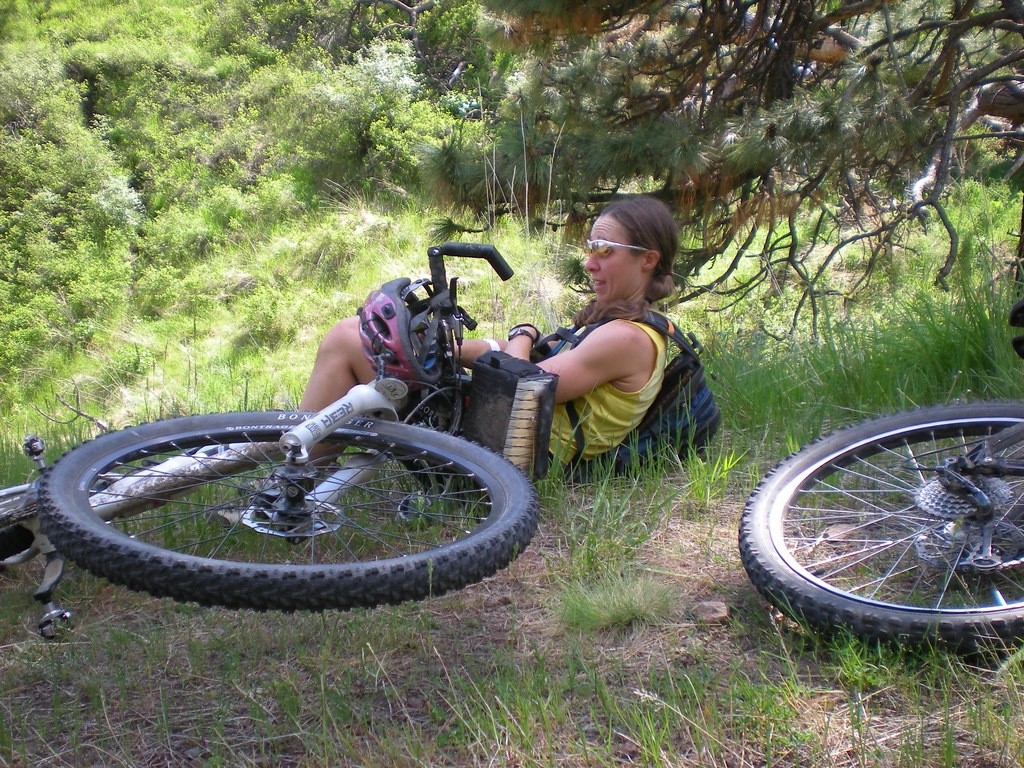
[216, 196, 678, 525]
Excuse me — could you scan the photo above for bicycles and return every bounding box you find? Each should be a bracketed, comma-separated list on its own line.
[0, 243, 545, 644]
[733, 70, 1024, 658]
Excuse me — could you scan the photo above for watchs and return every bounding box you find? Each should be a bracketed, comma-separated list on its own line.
[508, 327, 534, 347]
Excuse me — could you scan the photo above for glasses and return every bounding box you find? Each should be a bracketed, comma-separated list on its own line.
[586, 239, 649, 258]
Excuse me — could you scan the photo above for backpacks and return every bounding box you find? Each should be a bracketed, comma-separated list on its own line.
[538, 310, 720, 486]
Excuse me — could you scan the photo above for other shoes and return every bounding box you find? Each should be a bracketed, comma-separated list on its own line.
[238, 473, 316, 501]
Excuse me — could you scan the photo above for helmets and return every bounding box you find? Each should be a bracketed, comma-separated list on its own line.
[356, 277, 444, 385]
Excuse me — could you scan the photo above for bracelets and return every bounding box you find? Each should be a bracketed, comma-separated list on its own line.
[482, 338, 500, 352]
[510, 323, 539, 342]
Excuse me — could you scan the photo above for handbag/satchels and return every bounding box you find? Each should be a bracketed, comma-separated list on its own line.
[463, 350, 559, 486]
